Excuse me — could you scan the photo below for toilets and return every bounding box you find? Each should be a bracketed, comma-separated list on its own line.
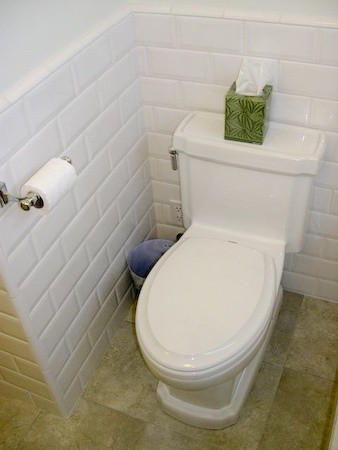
[133, 109, 325, 431]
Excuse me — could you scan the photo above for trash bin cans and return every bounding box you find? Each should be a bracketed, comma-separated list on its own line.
[127, 239, 176, 300]
